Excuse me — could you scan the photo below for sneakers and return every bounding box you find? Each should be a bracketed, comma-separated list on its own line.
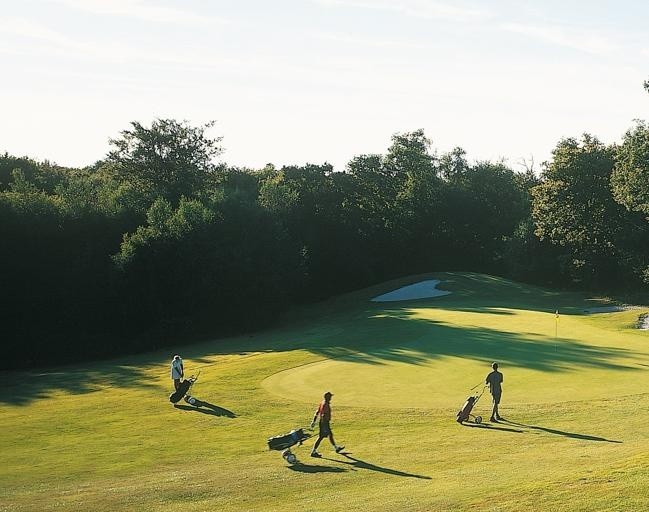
[490, 417, 497, 422]
[311, 452, 322, 457]
[336, 446, 345, 452]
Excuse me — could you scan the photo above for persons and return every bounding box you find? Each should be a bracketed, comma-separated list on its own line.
[171, 355, 183, 390]
[485, 362, 503, 421]
[311, 392, 345, 457]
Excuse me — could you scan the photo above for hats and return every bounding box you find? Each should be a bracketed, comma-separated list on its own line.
[325, 392, 334, 398]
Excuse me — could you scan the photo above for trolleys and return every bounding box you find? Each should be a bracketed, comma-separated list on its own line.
[457, 384, 488, 426]
[267, 421, 333, 464]
[170, 369, 200, 407]
